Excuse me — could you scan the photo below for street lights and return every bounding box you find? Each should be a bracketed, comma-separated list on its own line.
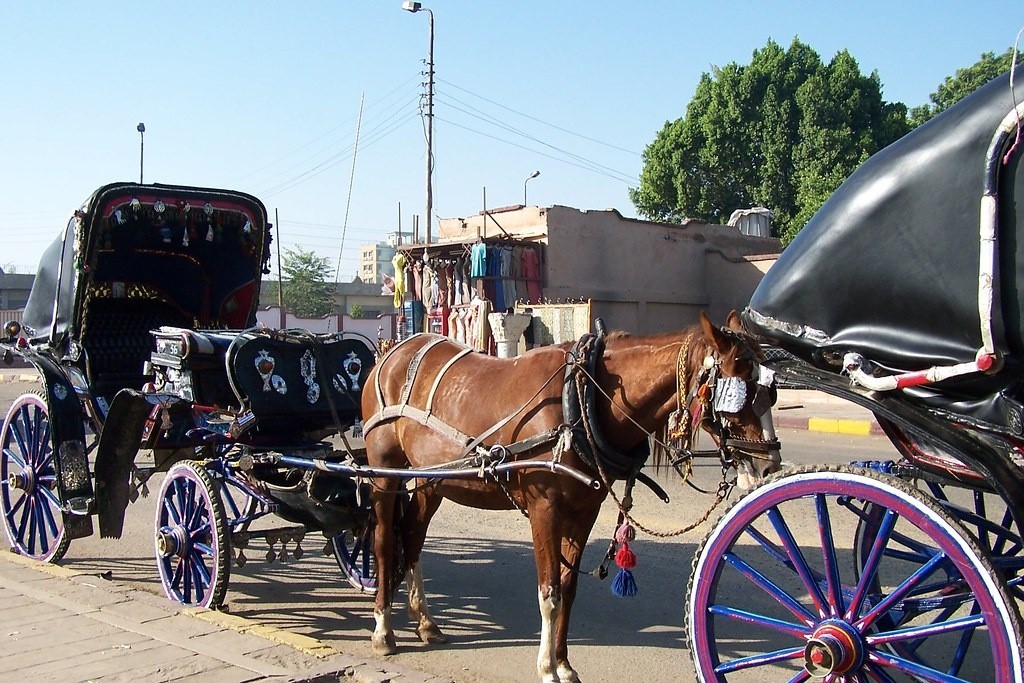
[402, 0, 435, 241]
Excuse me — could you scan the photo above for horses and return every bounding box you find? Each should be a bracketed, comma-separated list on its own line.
[359, 309, 784, 683]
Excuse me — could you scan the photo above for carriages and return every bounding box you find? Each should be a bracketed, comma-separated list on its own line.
[0, 181, 783, 683]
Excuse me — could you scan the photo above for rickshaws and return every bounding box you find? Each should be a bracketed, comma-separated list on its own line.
[685, 46, 1023, 682]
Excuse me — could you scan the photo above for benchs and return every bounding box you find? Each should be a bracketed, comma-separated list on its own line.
[145, 326, 312, 410]
[85, 280, 211, 392]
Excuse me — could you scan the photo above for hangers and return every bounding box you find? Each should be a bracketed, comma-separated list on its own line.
[394, 238, 538, 268]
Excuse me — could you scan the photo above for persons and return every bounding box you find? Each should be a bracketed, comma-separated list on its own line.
[391, 251, 406, 309]
[448, 309, 480, 349]
[403, 258, 477, 308]
